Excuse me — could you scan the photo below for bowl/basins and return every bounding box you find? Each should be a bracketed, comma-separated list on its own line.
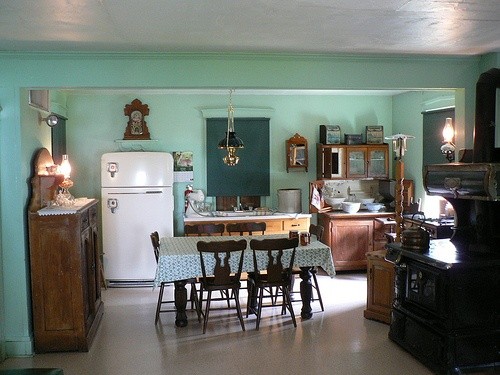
[366, 202, 382, 213]
[342, 202, 361, 213]
[328, 197, 346, 206]
[359, 197, 375, 210]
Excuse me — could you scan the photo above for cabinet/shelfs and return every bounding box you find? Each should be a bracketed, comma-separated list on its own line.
[395, 239, 498, 375]
[348, 145, 389, 178]
[366, 259, 395, 316]
[283, 218, 310, 232]
[373, 217, 397, 252]
[317, 212, 374, 271]
[317, 147, 346, 179]
[27, 199, 105, 353]
[185, 219, 283, 283]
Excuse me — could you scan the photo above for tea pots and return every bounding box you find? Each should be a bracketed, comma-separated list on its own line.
[401, 211, 430, 251]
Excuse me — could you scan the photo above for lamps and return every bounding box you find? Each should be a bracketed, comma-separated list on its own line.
[217, 89, 245, 167]
[439, 116, 456, 163]
[384, 133, 416, 243]
[59, 154, 74, 201]
[42, 115, 59, 127]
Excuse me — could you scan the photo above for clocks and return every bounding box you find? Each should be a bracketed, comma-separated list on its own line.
[122, 98, 151, 139]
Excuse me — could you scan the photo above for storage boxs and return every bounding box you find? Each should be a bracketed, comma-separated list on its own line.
[344, 133, 363, 145]
[365, 125, 385, 145]
[319, 124, 341, 145]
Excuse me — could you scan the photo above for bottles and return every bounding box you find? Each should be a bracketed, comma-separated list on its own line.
[300, 233, 308, 246]
[289, 230, 299, 243]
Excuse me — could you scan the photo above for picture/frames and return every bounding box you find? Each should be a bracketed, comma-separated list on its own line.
[28, 90, 50, 113]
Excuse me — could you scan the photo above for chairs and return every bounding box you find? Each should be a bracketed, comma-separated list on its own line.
[284, 224, 325, 314]
[150, 231, 202, 326]
[185, 223, 225, 313]
[226, 222, 266, 237]
[246, 237, 299, 331]
[197, 239, 247, 334]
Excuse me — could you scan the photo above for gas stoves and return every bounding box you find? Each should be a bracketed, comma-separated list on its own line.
[403, 217, 455, 239]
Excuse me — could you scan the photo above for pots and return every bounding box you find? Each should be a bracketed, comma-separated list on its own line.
[277, 188, 303, 213]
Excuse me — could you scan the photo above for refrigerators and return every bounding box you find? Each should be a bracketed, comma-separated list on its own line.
[101, 151, 175, 287]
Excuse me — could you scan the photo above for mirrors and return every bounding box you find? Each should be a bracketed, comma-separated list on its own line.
[473, 68, 500, 164]
[286, 132, 308, 173]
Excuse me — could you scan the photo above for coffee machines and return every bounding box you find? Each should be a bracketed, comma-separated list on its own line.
[185, 189, 210, 218]
